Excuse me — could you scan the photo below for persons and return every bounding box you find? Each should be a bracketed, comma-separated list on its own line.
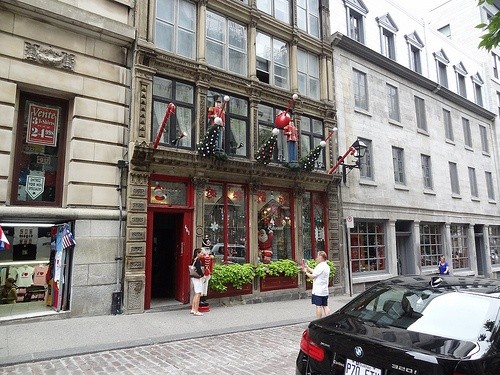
[191, 248, 205, 316]
[303, 251, 331, 318]
[284, 119, 298, 163]
[2, 278, 17, 304]
[208, 98, 225, 151]
[438, 257, 449, 275]
[200, 238, 213, 307]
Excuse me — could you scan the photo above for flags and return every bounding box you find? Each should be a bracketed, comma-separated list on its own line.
[0, 228, 10, 250]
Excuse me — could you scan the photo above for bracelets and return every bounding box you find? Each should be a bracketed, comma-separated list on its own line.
[305, 271, 307, 274]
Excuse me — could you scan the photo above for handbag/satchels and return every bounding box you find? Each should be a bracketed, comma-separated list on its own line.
[188, 257, 204, 278]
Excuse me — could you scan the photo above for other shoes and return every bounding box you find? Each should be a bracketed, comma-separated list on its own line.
[190, 310, 205, 316]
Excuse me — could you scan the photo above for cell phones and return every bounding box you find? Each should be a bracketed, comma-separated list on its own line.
[302, 259, 304, 265]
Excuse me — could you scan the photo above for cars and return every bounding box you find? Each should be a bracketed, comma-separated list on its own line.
[295, 273, 500, 375]
